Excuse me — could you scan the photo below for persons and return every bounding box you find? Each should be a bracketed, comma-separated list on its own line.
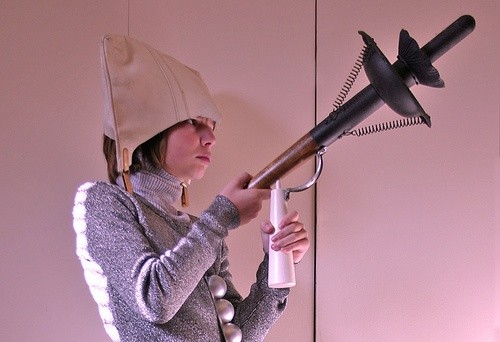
[71, 33, 311, 342]
